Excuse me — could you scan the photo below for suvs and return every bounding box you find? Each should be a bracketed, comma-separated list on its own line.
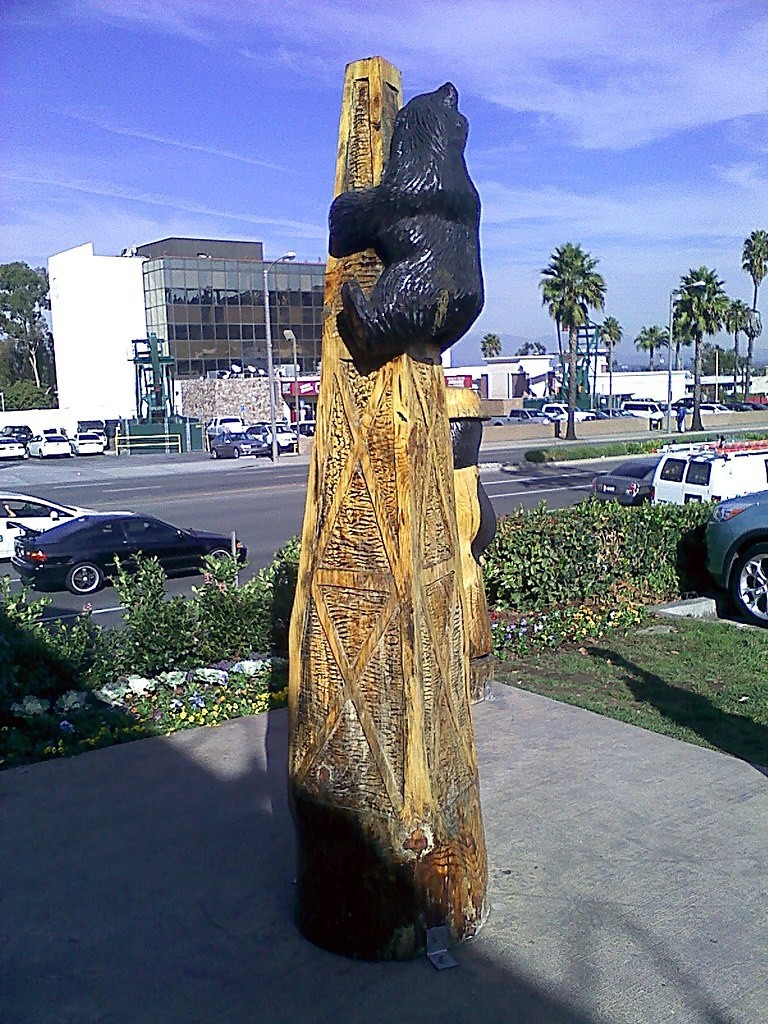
[0, 425, 33, 445]
[649, 449, 767, 510]
[542, 403, 596, 422]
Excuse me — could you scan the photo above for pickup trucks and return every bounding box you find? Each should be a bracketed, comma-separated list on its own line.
[488, 409, 553, 426]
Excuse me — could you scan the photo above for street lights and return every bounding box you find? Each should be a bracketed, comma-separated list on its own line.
[263, 252, 297, 463]
[667, 281, 707, 433]
[283, 330, 301, 440]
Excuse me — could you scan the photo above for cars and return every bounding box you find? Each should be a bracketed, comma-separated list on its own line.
[210, 431, 269, 460]
[26, 434, 71, 459]
[246, 424, 300, 456]
[69, 433, 104, 456]
[589, 457, 660, 507]
[0, 437, 26, 458]
[88, 429, 108, 447]
[706, 491, 768, 629]
[0, 491, 100, 559]
[204, 417, 252, 439]
[587, 397, 767, 419]
[11, 513, 248, 595]
[291, 420, 316, 438]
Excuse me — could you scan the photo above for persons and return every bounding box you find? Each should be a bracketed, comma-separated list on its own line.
[4, 504, 17, 517]
[675, 406, 685, 433]
